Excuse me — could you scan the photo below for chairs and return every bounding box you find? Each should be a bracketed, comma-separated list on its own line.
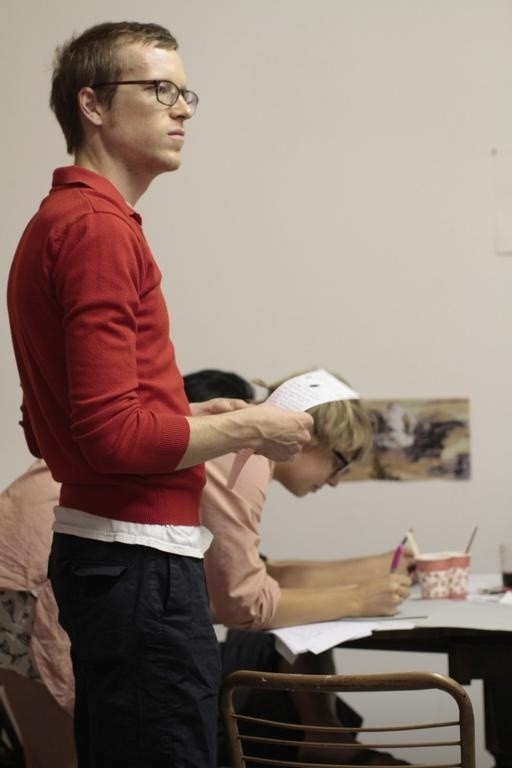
[217, 665, 477, 768]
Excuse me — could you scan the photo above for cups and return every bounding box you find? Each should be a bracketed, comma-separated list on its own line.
[414, 554, 450, 600]
[499, 543, 512, 590]
[439, 551, 471, 599]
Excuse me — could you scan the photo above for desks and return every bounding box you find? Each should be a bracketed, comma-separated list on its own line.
[231, 574, 512, 768]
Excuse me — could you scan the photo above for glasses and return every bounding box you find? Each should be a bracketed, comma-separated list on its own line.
[313, 428, 355, 480]
[87, 78, 199, 118]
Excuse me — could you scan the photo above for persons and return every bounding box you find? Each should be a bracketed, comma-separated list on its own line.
[179, 365, 420, 588]
[0, 365, 414, 767]
[4, 19, 317, 766]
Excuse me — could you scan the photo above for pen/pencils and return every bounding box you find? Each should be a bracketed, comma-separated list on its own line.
[390, 545, 403, 574]
[400, 528, 413, 545]
[464, 523, 478, 553]
